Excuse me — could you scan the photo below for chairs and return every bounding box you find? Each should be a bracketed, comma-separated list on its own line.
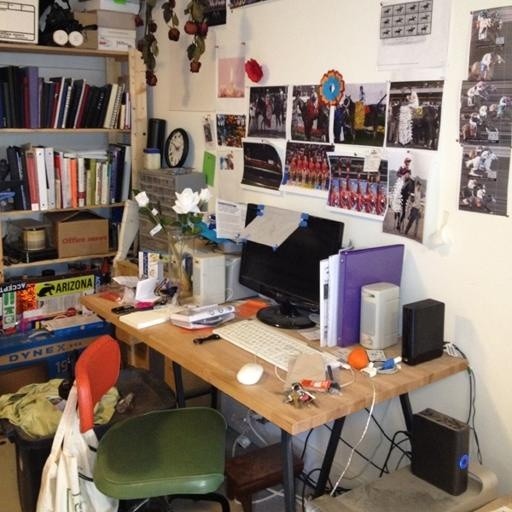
[62, 335, 228, 511]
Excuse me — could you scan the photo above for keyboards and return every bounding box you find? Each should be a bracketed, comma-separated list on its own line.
[212, 317, 343, 378]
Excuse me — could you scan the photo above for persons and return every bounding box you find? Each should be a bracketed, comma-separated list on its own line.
[293, 87, 329, 143]
[466, 147, 493, 207]
[285, 145, 328, 189]
[260, 87, 286, 130]
[471, 11, 503, 74]
[391, 158, 423, 239]
[469, 83, 508, 139]
[356, 85, 368, 101]
[406, 88, 419, 108]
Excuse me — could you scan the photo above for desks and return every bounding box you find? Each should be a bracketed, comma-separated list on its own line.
[79, 288, 468, 512]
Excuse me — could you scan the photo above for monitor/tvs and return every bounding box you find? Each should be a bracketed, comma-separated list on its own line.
[238, 202, 344, 331]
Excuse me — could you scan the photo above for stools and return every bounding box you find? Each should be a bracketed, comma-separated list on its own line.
[225, 443, 304, 512]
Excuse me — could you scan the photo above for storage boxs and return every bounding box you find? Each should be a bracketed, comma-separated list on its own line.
[44, 210, 109, 258]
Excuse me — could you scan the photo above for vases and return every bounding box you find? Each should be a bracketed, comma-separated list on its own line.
[166, 234, 199, 298]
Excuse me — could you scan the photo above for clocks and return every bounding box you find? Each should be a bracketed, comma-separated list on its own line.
[165, 128, 189, 168]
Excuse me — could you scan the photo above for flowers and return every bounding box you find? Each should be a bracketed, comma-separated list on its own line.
[133, 187, 211, 252]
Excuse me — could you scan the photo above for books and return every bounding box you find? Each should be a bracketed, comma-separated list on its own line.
[118, 307, 184, 329]
[0, 64, 132, 211]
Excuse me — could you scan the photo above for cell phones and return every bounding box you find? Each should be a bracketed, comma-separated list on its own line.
[111, 304, 134, 315]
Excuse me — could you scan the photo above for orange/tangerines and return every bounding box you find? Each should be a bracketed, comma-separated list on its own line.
[349, 346, 369, 368]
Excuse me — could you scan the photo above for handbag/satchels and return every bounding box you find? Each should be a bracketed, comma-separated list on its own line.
[36, 379, 119, 512]
[0, 378, 119, 442]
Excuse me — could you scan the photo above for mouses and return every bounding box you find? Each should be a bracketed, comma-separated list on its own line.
[236, 362, 264, 384]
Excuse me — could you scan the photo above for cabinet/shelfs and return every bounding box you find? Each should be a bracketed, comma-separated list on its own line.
[0, 43, 148, 366]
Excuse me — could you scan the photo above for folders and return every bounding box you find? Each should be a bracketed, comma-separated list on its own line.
[31, 146, 48, 211]
[25, 151, 39, 210]
[7, 145, 31, 211]
[40, 144, 55, 209]
[337, 245, 404, 347]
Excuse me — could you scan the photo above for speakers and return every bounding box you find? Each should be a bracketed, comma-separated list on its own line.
[192, 253, 226, 307]
[360, 282, 401, 350]
